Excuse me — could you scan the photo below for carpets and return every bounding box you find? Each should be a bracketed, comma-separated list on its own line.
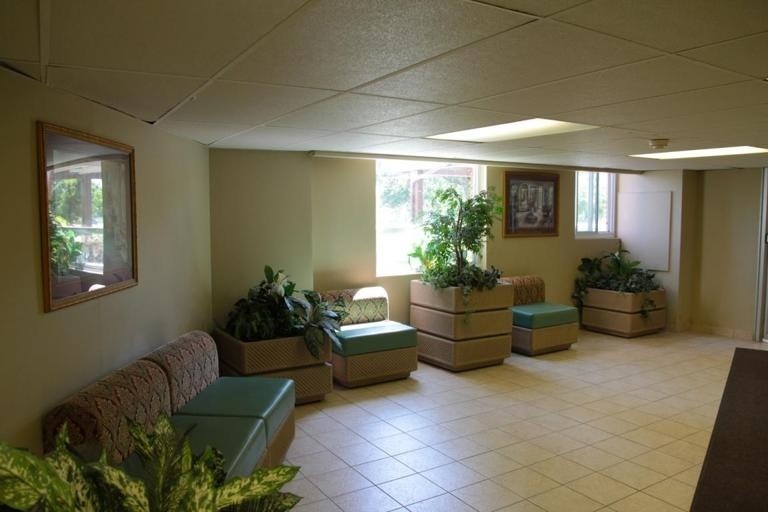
[689, 347, 767, 511]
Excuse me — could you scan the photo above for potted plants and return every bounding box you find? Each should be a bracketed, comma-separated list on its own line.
[409, 185, 516, 374]
[575, 244, 668, 339]
[213, 265, 333, 407]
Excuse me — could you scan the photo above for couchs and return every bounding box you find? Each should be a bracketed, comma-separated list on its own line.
[40, 330, 298, 488]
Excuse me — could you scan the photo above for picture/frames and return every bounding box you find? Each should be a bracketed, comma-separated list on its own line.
[503, 170, 561, 237]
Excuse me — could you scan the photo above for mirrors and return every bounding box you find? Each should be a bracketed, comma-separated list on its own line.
[34, 118, 139, 312]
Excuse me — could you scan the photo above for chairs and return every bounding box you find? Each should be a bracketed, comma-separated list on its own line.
[501, 274, 580, 357]
[320, 284, 418, 391]
